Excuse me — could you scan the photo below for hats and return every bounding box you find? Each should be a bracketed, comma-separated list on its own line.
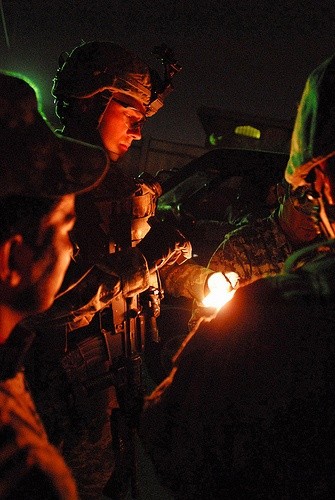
[1, 72, 111, 197]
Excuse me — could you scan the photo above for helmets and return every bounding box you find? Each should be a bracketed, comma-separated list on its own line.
[54, 41, 181, 127]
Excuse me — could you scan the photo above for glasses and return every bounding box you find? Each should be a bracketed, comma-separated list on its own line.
[110, 94, 147, 129]
[286, 184, 320, 216]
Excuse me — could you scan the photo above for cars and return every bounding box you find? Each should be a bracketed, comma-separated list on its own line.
[138, 144, 290, 268]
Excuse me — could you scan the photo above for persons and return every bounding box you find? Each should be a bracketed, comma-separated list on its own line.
[187, 172, 321, 327]
[0, 73, 108, 500]
[36, 41, 192, 500]
[148, 53, 334, 499]
